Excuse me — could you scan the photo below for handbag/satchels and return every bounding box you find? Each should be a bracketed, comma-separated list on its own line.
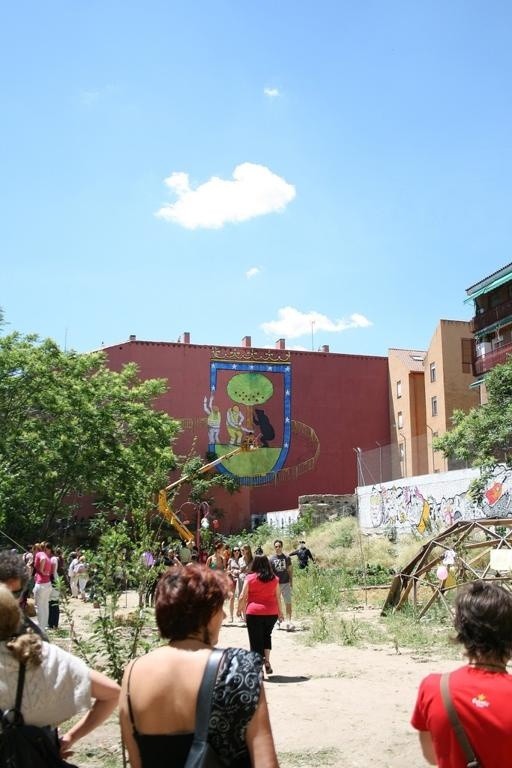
[185, 741, 231, 768]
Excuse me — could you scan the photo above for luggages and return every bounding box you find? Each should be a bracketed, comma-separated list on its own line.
[49, 600, 59, 628]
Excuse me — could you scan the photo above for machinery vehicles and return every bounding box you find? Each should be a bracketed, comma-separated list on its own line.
[153, 428, 264, 566]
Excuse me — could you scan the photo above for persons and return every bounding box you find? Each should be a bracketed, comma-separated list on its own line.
[118, 563, 279, 768]
[114, 539, 315, 681]
[412, 582, 512, 768]
[0, 583, 121, 768]
[1, 541, 109, 643]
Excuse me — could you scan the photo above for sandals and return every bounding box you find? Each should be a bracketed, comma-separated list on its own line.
[265, 660, 272, 674]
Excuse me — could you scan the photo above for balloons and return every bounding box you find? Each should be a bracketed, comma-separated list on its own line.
[437, 566, 449, 581]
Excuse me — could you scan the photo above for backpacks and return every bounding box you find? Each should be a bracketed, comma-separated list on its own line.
[0, 661, 60, 768]
